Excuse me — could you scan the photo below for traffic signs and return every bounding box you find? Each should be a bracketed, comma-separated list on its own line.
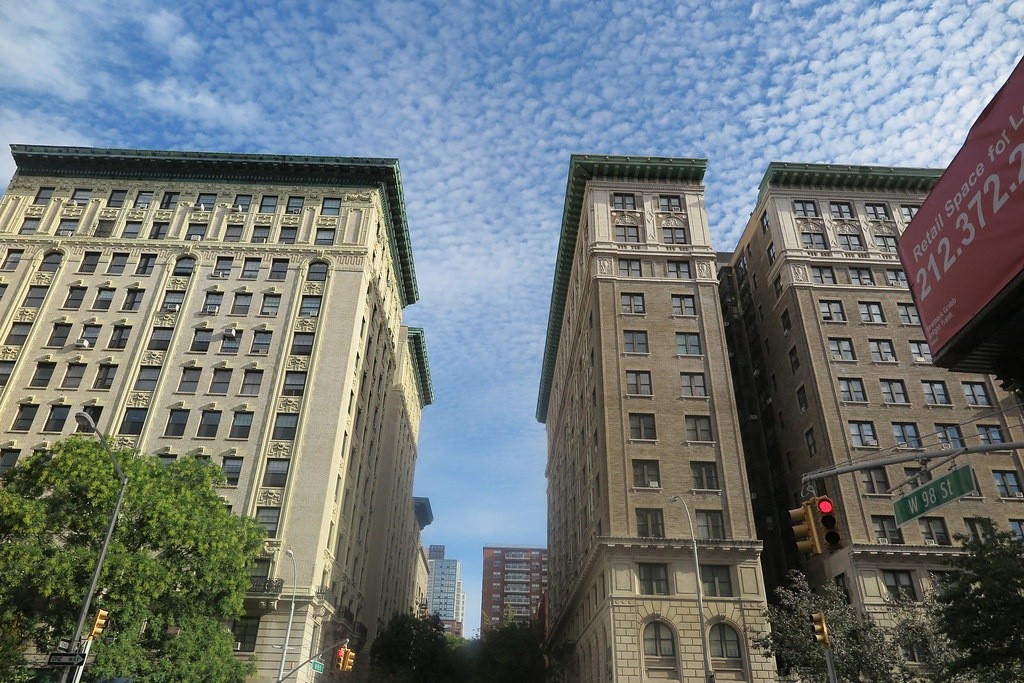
[48, 653, 87, 668]
[58, 638, 80, 653]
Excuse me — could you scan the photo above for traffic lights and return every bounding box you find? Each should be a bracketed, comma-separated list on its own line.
[88, 609, 110, 638]
[814, 496, 845, 551]
[789, 502, 822, 552]
[336, 647, 344, 671]
[343, 649, 356, 672]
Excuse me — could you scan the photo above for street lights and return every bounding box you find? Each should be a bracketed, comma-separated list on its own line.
[810, 610, 830, 646]
[708, 671, 715, 683]
[275, 549, 298, 683]
[56, 410, 129, 683]
[667, 495, 716, 683]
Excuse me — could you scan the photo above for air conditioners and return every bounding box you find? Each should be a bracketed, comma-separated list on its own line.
[167, 303, 180, 312]
[74, 338, 89, 347]
[190, 235, 202, 242]
[878, 537, 888, 544]
[211, 271, 222, 277]
[924, 539, 935, 545]
[224, 328, 236, 337]
[207, 305, 219, 313]
[1014, 492, 1023, 498]
